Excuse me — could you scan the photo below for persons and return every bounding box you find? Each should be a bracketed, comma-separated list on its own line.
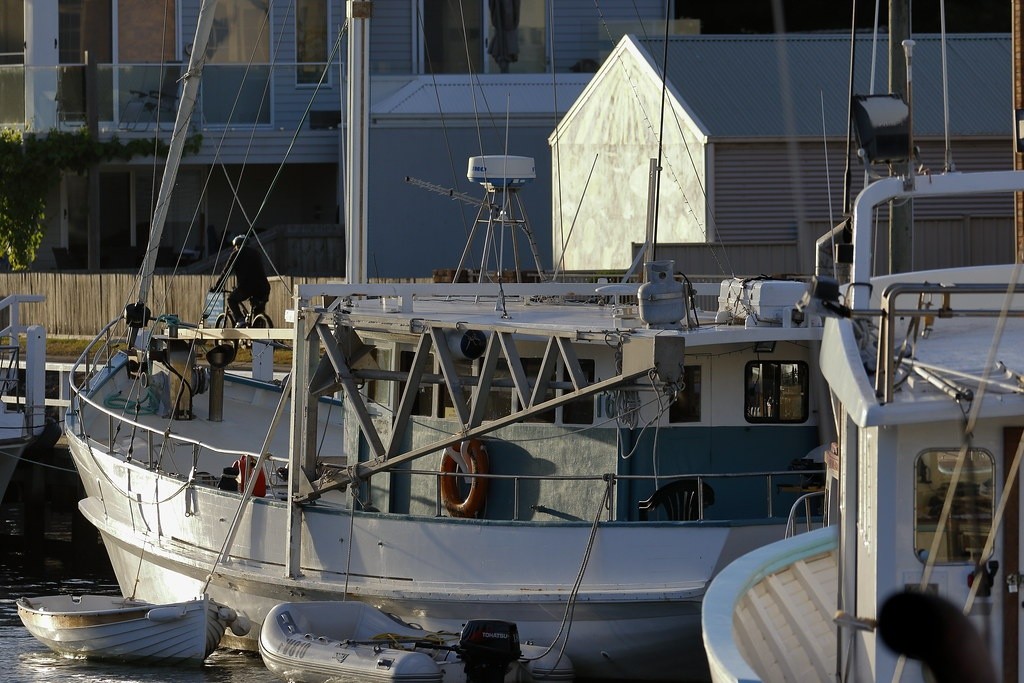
[215, 234, 271, 328]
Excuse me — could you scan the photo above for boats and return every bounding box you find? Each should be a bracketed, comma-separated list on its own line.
[15, 593, 251, 668]
[258, 599, 574, 683]
[54, 281, 872, 683]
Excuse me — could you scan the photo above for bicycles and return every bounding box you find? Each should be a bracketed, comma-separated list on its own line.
[209, 288, 274, 329]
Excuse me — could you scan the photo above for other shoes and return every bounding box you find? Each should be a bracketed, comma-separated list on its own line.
[235, 322, 246, 328]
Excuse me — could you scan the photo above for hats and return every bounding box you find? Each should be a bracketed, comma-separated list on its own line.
[232, 235, 250, 246]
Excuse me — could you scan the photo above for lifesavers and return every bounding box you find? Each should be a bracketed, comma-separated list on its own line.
[438, 435, 489, 518]
